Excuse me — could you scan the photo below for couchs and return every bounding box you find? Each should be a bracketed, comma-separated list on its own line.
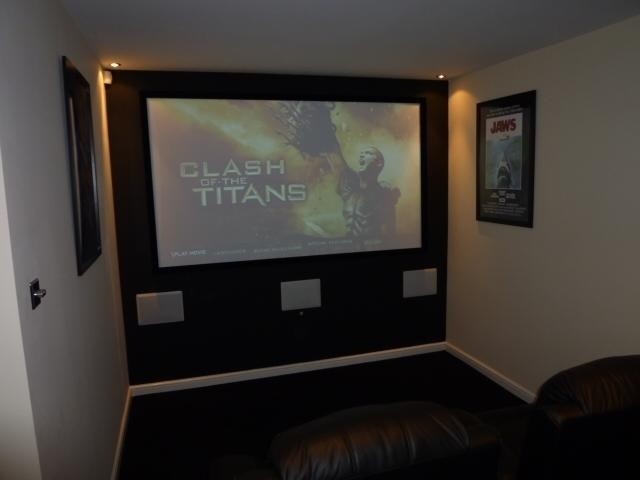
[262, 354, 640, 479]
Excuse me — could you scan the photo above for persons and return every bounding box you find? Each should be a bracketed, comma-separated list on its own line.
[322, 126, 400, 238]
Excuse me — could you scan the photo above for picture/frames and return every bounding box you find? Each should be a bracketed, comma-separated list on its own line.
[61, 56, 104, 275]
[475, 88, 537, 230]
